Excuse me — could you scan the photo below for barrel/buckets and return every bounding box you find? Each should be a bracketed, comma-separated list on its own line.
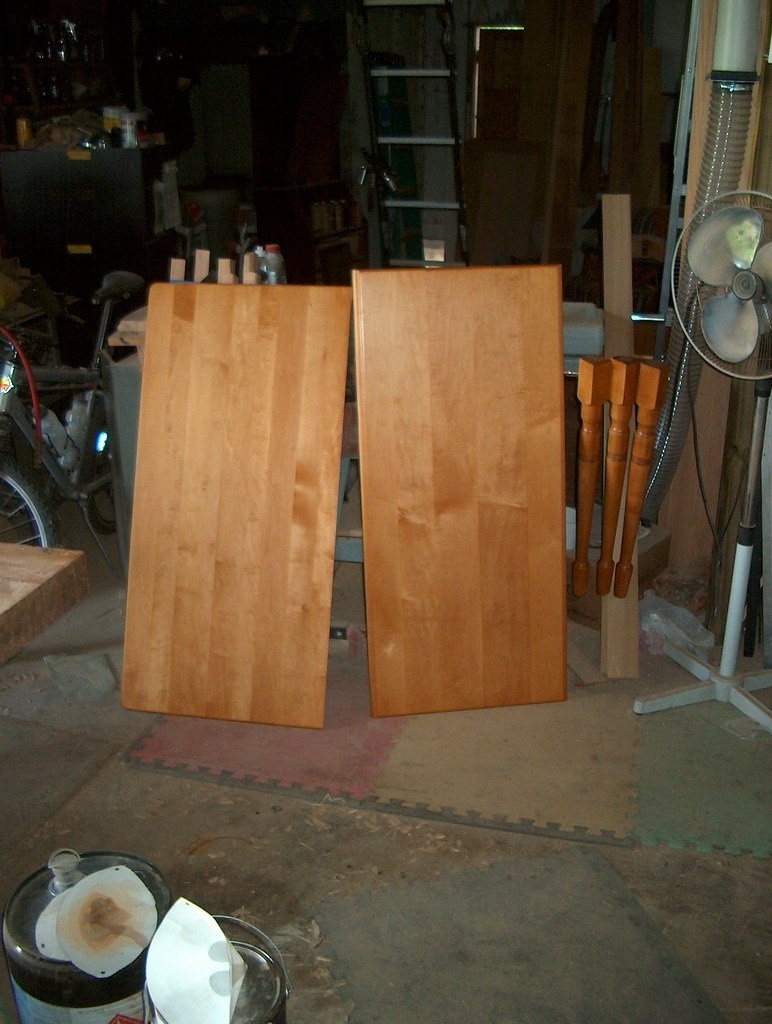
[3, 849, 173, 1024]
[143, 913, 291, 1024]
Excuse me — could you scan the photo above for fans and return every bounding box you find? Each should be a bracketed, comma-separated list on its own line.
[634, 190, 772, 733]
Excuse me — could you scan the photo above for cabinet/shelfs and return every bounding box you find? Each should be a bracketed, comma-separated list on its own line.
[269, 178, 364, 283]
[0, 61, 109, 120]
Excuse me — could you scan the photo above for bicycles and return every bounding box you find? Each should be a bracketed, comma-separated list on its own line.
[1, 271, 156, 549]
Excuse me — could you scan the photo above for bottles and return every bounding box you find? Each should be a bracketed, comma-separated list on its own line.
[62, 390, 91, 451]
[311, 199, 347, 232]
[31, 404, 80, 472]
[265, 244, 288, 285]
